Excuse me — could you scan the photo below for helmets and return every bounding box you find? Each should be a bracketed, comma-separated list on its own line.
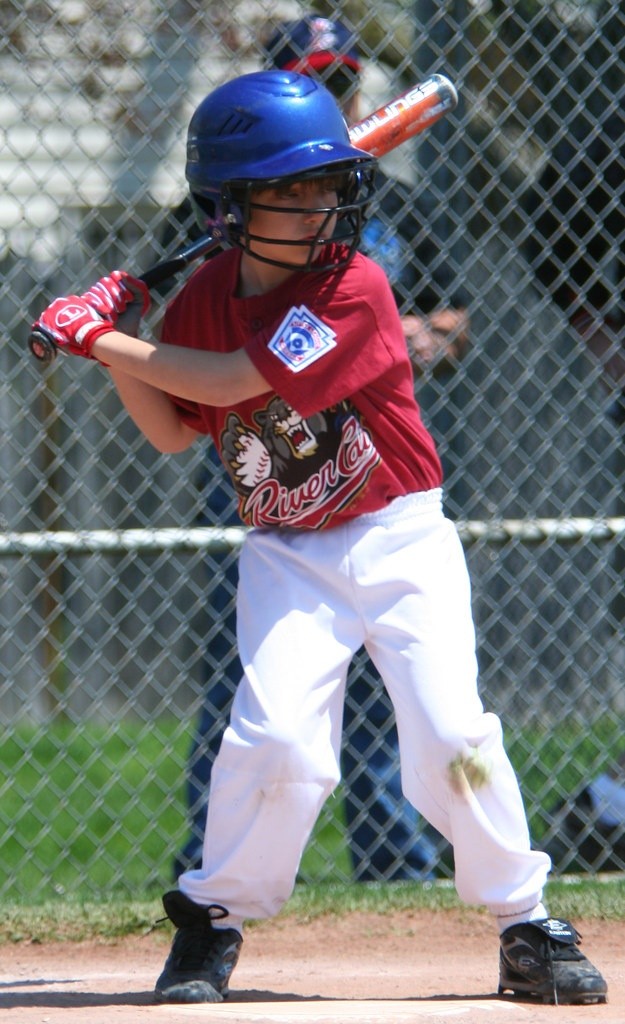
[185, 70, 377, 238]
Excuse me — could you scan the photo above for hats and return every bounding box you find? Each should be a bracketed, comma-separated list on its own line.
[261, 15, 360, 75]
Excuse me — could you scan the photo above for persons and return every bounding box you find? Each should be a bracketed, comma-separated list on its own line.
[32, 71, 610, 1007]
[171, 18, 466, 885]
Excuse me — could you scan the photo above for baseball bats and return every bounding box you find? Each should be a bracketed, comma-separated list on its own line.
[25, 73, 458, 364]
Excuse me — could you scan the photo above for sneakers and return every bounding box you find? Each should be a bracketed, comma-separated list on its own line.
[498, 918, 608, 1005]
[155, 890, 243, 1004]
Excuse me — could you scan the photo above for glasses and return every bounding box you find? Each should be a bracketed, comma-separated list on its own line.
[320, 70, 354, 97]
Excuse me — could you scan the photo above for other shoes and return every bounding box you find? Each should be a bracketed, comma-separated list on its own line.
[353, 842, 460, 883]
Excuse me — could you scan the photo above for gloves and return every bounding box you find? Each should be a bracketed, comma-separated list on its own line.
[80, 270, 152, 366]
[32, 295, 117, 358]
